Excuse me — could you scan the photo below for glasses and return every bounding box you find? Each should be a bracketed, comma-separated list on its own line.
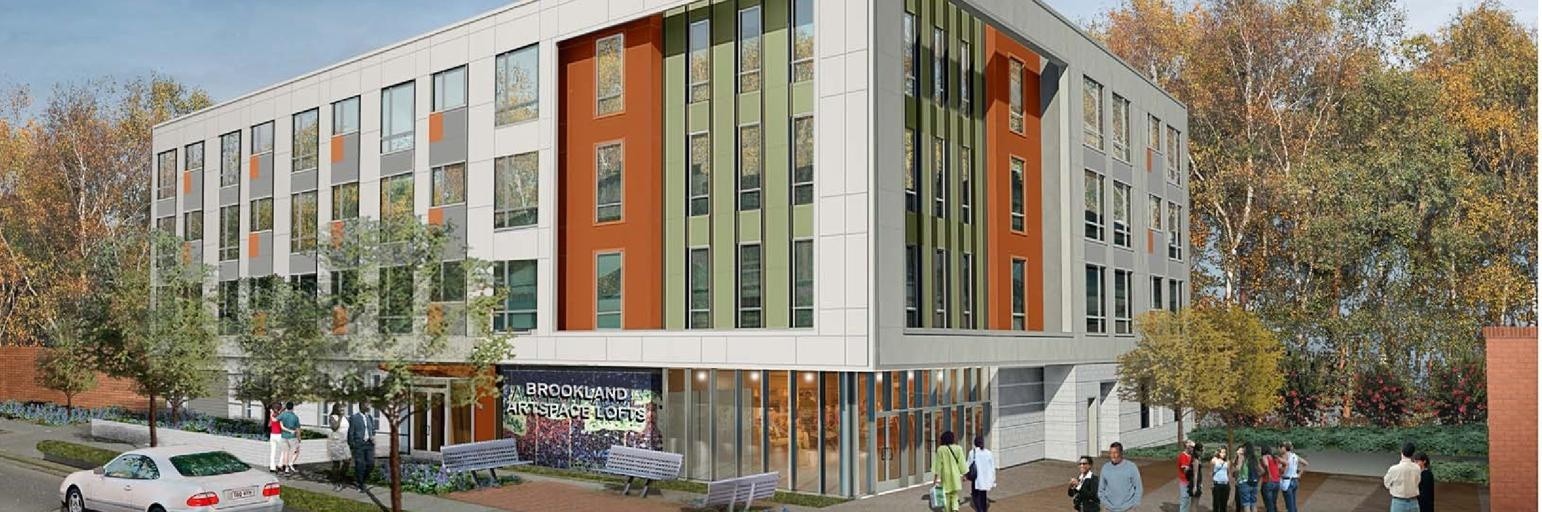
[1076, 461, 1091, 466]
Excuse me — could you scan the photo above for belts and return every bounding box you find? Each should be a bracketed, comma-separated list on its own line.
[1393, 496, 1418, 500]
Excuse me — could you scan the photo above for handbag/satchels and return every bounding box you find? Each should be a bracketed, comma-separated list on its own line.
[967, 448, 977, 481]
[929, 484, 945, 509]
[1279, 477, 1291, 493]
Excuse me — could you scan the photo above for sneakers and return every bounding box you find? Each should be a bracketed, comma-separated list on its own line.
[267, 465, 302, 477]
[331, 480, 370, 493]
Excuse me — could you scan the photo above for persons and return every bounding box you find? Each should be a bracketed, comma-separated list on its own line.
[1096, 442, 1143, 512]
[1068, 456, 1100, 512]
[1413, 452, 1435, 512]
[963, 435, 996, 512]
[268, 402, 297, 473]
[347, 400, 376, 493]
[931, 430, 969, 512]
[269, 402, 301, 473]
[799, 389, 817, 434]
[327, 403, 352, 492]
[1383, 442, 1422, 512]
[1177, 439, 1309, 512]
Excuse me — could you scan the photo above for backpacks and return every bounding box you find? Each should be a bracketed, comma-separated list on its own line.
[1245, 457, 1261, 485]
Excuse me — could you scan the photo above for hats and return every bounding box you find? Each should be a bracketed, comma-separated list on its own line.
[1283, 441, 1296, 450]
